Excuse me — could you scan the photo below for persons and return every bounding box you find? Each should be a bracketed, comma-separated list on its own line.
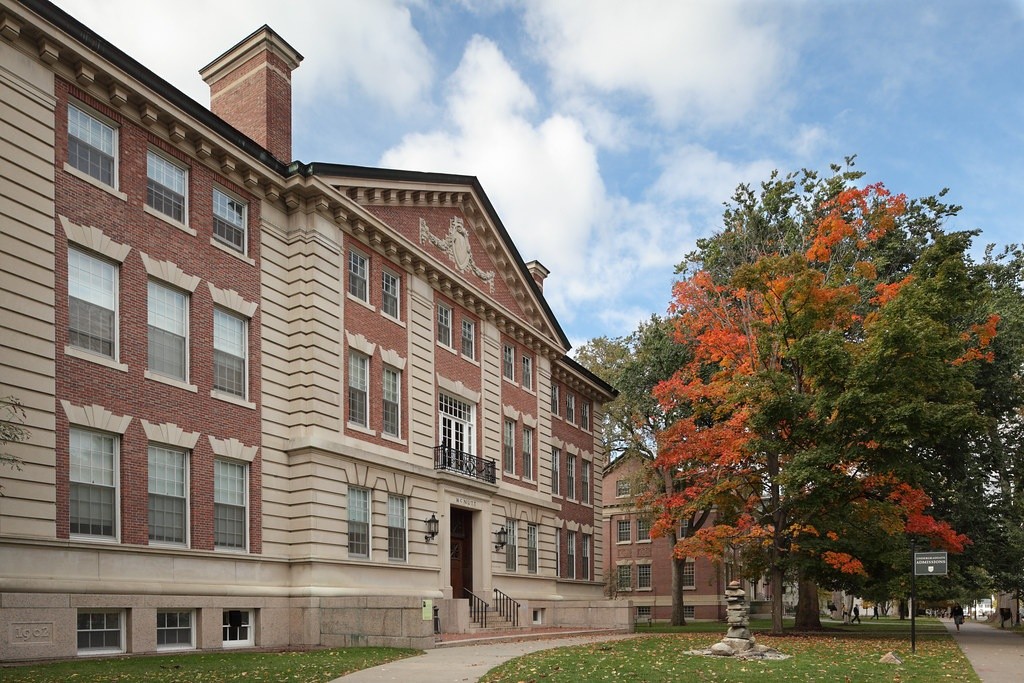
[851, 603, 861, 624]
[841, 604, 846, 621]
[870, 605, 879, 620]
[830, 603, 836, 618]
[950, 602, 964, 633]
[937, 608, 945, 618]
